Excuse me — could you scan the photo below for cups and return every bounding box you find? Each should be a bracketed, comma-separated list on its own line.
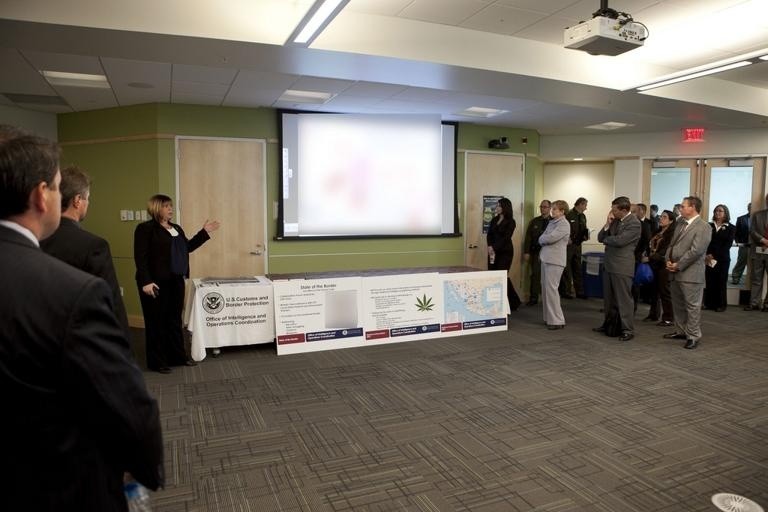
[489, 251, 496, 264]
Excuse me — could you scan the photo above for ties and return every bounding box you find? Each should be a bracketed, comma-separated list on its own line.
[677, 222, 688, 240]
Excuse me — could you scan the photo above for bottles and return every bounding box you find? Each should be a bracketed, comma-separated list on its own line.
[124, 477, 154, 511]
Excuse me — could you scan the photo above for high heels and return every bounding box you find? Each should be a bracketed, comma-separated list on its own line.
[549, 324, 564, 330]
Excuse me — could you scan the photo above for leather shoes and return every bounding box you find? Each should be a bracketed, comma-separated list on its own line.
[684, 339, 697, 349]
[618, 333, 633, 341]
[526, 298, 537, 306]
[150, 366, 171, 374]
[642, 317, 657, 322]
[182, 360, 197, 366]
[716, 306, 727, 312]
[702, 305, 711, 310]
[562, 294, 574, 299]
[663, 332, 685, 338]
[576, 294, 588, 299]
[592, 325, 604, 333]
[743, 305, 759, 311]
[658, 320, 671, 326]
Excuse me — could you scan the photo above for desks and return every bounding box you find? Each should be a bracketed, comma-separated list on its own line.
[191, 266, 489, 358]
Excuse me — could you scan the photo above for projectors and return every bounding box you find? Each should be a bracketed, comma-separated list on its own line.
[563, 15, 646, 56]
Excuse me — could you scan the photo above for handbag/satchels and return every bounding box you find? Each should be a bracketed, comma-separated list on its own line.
[603, 308, 621, 336]
[634, 259, 654, 286]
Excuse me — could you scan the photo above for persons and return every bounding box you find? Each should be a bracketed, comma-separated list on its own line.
[732, 203, 752, 285]
[701, 205, 737, 312]
[134, 193, 221, 374]
[38, 167, 135, 354]
[487, 198, 521, 312]
[558, 198, 588, 300]
[538, 200, 571, 331]
[743, 194, 768, 313]
[663, 196, 713, 350]
[523, 200, 553, 306]
[0, 136, 166, 512]
[592, 196, 682, 341]
